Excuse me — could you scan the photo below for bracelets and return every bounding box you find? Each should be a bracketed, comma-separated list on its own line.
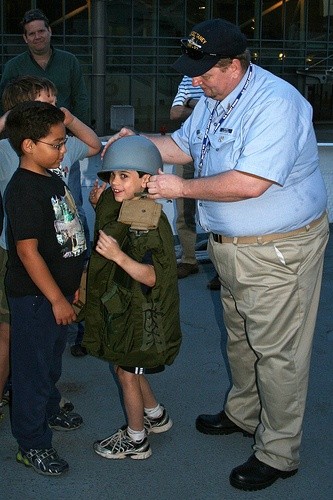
[186, 98, 192, 109]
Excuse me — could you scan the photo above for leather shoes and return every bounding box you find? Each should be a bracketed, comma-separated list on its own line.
[229, 455, 298, 492]
[196, 410, 255, 438]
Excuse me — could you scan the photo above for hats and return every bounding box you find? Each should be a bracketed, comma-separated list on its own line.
[170, 19, 247, 76]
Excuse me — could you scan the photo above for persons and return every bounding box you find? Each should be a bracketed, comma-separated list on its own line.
[1, 75, 180, 478]
[171, 70, 224, 288]
[3, 11, 93, 138]
[101, 17, 331, 491]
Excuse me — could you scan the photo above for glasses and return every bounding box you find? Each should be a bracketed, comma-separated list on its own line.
[36, 138, 68, 150]
[178, 38, 240, 61]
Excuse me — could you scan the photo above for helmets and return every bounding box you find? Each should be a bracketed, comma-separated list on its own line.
[97, 134, 164, 183]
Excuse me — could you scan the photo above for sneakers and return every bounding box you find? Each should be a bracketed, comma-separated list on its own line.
[49, 413, 82, 431]
[93, 424, 153, 460]
[143, 403, 173, 433]
[16, 447, 70, 475]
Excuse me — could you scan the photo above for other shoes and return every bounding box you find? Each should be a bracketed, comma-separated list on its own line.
[176, 262, 199, 279]
[208, 275, 222, 291]
